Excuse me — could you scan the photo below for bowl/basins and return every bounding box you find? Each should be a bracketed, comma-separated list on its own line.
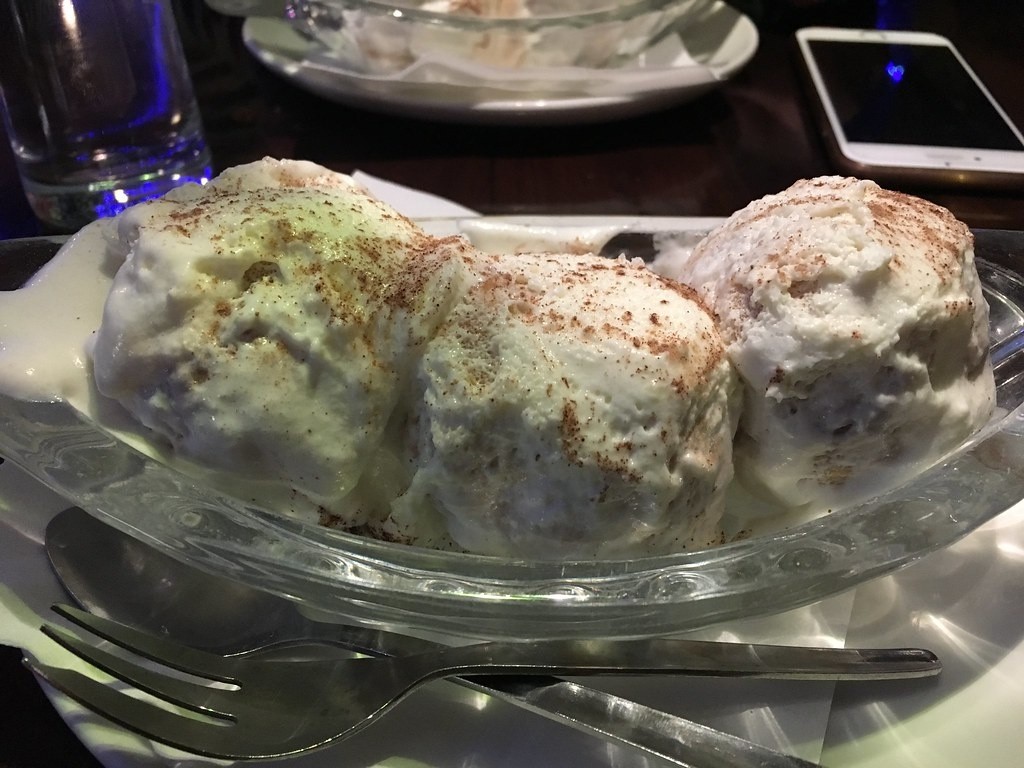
[319, 0, 718, 81]
[0, 226, 1024, 634]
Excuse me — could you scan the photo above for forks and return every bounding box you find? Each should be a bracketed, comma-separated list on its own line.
[22, 605, 944, 761]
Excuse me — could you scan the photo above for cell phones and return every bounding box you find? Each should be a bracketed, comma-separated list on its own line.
[791, 26, 1024, 196]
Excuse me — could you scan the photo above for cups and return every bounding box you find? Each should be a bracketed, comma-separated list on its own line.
[0, 0, 213, 224]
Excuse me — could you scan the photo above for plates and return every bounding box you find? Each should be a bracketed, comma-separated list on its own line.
[242, 0, 758, 110]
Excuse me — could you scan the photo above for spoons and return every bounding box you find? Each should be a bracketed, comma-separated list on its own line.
[45, 507, 822, 768]
